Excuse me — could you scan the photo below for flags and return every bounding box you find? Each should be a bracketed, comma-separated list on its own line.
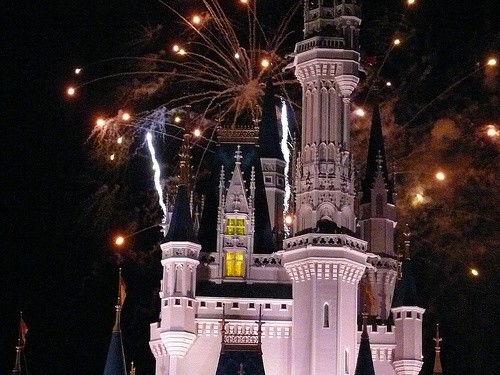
[118, 276, 126, 307]
[20, 318, 28, 345]
